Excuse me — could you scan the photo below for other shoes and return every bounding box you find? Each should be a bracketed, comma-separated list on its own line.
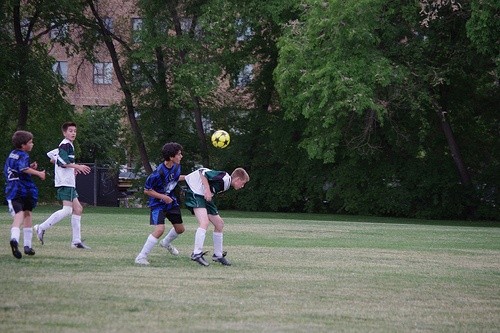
[24, 246, 35, 255]
[134, 257, 152, 265]
[10, 238, 22, 258]
[160, 239, 179, 256]
[71, 242, 91, 250]
[33, 224, 45, 244]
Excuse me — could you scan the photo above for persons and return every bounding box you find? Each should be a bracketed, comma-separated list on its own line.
[4, 131, 45, 259]
[184, 168, 249, 267]
[34, 122, 92, 249]
[135, 143, 203, 265]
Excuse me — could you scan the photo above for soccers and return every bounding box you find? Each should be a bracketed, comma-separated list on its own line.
[211, 130, 231, 149]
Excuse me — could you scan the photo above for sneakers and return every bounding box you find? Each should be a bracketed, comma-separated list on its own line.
[191, 250, 210, 266]
[212, 251, 231, 266]
